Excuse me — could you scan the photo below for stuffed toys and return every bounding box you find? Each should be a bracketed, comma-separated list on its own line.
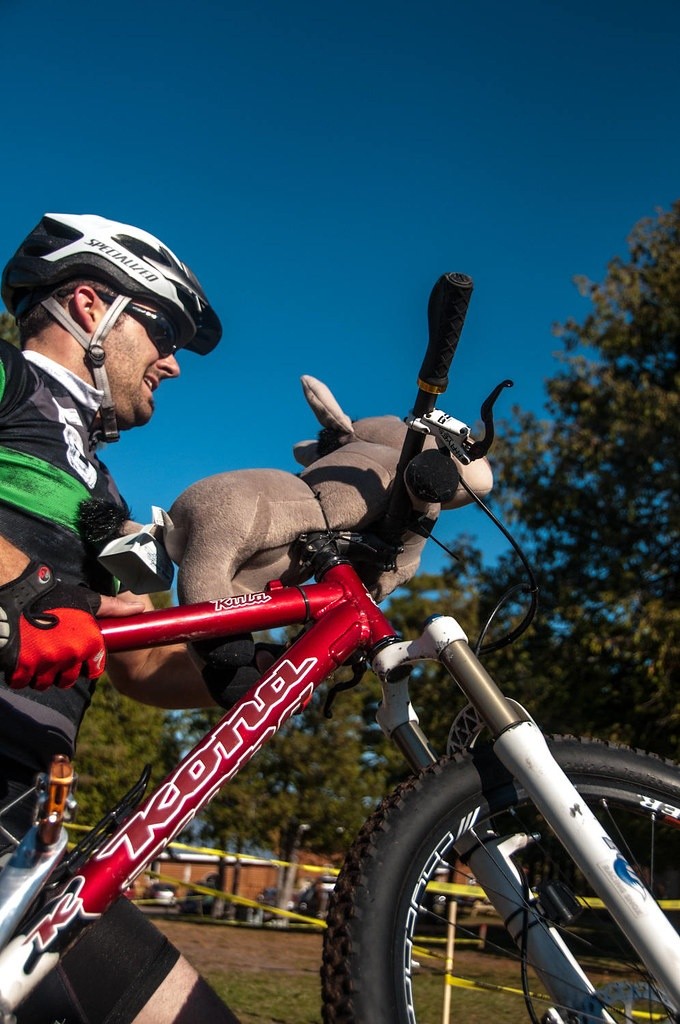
[147, 375, 493, 673]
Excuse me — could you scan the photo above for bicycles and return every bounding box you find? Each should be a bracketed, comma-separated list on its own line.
[1, 268, 680, 1024]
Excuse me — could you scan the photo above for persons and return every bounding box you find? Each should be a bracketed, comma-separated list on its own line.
[0, 210, 291, 1024]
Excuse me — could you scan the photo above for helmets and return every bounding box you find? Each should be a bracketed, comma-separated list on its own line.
[0, 213, 222, 355]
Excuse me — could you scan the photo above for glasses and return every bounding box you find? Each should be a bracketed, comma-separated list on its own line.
[57, 288, 177, 359]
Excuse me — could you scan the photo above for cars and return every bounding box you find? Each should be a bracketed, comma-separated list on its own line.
[141, 882, 177, 902]
[255, 887, 296, 912]
